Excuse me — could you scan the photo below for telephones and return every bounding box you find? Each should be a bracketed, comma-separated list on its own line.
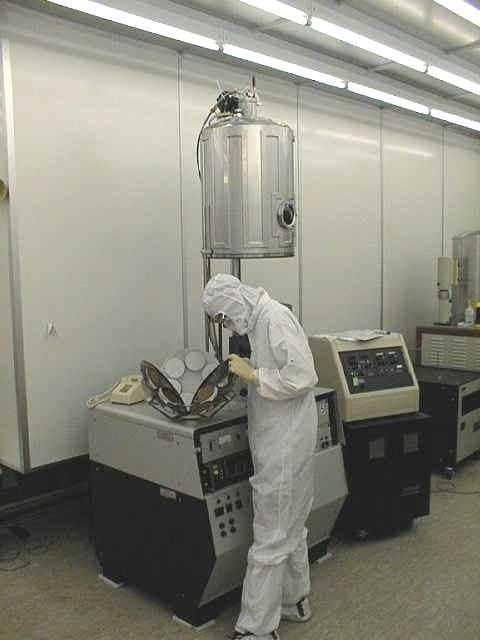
[111, 374, 144, 405]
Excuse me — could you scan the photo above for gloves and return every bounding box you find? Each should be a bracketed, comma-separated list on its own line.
[228, 351, 258, 387]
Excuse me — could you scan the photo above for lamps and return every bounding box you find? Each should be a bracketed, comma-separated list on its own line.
[49, 0, 480, 138]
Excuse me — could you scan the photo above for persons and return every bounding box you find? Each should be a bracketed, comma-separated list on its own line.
[199, 269, 320, 639]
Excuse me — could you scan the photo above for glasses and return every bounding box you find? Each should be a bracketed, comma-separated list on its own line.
[213, 314, 228, 324]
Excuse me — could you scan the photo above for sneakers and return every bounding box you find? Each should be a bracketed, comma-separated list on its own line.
[226, 628, 281, 640]
[280, 596, 314, 623]
